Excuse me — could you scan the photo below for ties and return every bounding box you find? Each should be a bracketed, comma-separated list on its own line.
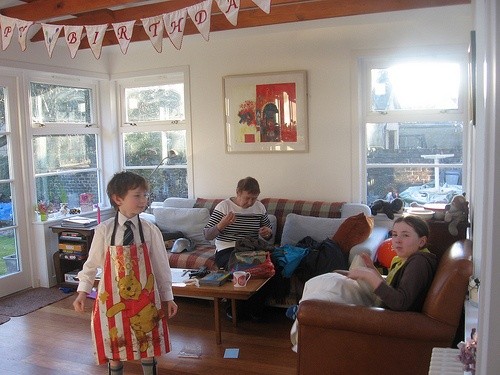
[123, 221, 134, 246]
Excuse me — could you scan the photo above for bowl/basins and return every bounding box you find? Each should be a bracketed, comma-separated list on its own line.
[424, 204, 448, 220]
[408, 209, 435, 220]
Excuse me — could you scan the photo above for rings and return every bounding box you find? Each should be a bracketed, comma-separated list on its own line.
[267, 235, 268, 236]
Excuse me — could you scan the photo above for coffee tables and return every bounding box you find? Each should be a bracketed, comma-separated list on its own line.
[166, 268, 272, 344]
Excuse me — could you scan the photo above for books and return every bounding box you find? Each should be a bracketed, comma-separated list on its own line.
[58, 216, 98, 261]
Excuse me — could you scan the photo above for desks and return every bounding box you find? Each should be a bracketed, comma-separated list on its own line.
[49, 222, 102, 289]
[423, 220, 468, 253]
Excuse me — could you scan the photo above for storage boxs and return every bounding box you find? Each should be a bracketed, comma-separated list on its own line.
[56, 244, 84, 283]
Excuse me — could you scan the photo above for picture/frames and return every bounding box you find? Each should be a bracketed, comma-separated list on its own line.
[221, 69, 309, 154]
[468, 30, 476, 125]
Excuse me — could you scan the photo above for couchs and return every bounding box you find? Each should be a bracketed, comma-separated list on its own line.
[141, 197, 388, 310]
[296, 239, 472, 375]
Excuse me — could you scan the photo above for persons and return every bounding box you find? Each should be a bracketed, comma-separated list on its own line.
[73, 172, 178, 358]
[203, 177, 276, 321]
[287, 216, 437, 320]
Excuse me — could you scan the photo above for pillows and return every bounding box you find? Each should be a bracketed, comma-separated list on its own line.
[333, 214, 375, 252]
[153, 206, 211, 244]
[281, 212, 346, 245]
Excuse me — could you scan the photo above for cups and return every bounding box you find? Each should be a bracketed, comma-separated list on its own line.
[233, 271, 251, 288]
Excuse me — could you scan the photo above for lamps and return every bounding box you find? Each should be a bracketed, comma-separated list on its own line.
[149, 150, 177, 178]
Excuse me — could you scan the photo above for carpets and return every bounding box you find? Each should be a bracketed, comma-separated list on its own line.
[0, 287, 74, 326]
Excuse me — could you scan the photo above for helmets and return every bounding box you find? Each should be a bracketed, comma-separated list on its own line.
[170, 238, 191, 253]
